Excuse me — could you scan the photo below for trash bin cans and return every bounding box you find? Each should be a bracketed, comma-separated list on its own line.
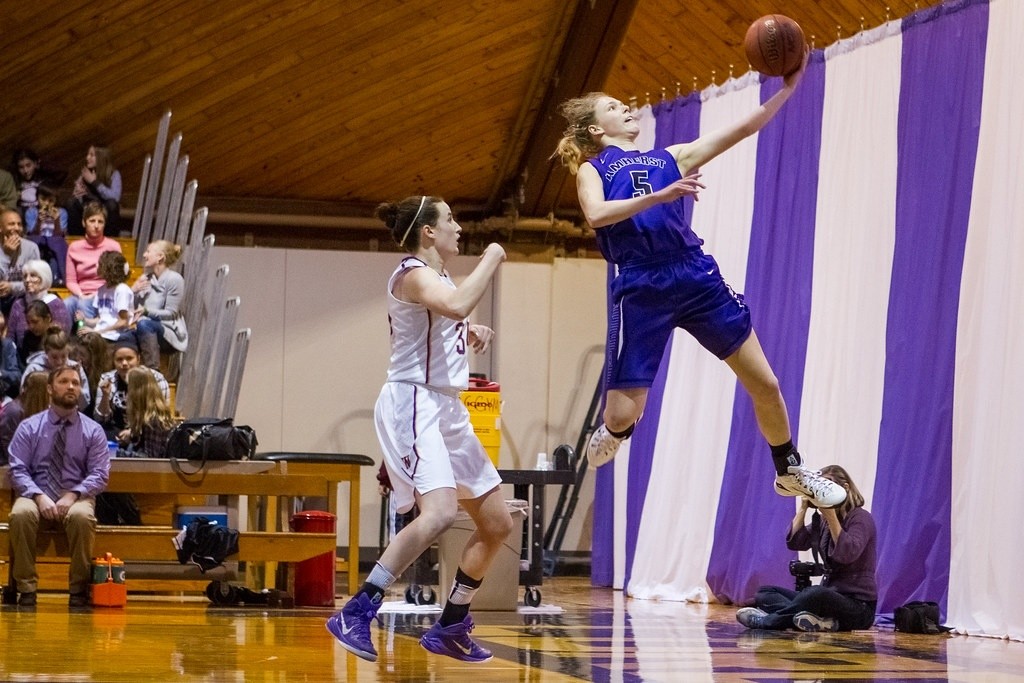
[438, 500, 529, 610]
[290, 511, 338, 606]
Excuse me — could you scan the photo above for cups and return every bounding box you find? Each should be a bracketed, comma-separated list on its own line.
[108, 443, 119, 458]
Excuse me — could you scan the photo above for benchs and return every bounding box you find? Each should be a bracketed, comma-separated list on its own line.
[49, 236, 148, 299]
[0, 454, 337, 608]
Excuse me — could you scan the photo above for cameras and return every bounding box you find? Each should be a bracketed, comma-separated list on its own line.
[789, 559, 823, 592]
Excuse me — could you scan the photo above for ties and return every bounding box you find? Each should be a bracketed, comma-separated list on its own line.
[44, 419, 68, 503]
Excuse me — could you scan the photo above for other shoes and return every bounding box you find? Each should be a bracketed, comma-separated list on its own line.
[19, 592, 36, 606]
[69, 591, 89, 609]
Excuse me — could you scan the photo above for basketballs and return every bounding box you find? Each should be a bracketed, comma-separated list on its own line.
[744, 13, 807, 78]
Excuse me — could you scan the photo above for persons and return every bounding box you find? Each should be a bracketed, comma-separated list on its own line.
[8, 367, 111, 604]
[378, 462, 403, 544]
[0, 144, 189, 524]
[736, 465, 877, 632]
[325, 196, 512, 662]
[559, 45, 848, 508]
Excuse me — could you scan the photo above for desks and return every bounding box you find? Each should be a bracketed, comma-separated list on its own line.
[244, 452, 376, 592]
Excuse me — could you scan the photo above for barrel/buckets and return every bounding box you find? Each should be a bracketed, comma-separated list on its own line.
[289, 511, 337, 605]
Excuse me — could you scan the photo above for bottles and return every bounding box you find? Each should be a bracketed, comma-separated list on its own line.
[77, 317, 86, 337]
[537, 452, 546, 466]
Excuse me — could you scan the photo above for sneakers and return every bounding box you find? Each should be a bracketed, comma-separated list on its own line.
[735, 627, 764, 649]
[587, 412, 643, 467]
[793, 611, 839, 632]
[325, 592, 384, 662]
[418, 614, 493, 663]
[797, 632, 838, 649]
[774, 452, 848, 509]
[736, 607, 770, 629]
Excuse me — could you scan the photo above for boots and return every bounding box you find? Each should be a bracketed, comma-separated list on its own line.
[140, 334, 160, 372]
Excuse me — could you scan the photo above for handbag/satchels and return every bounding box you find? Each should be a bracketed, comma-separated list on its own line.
[172, 517, 239, 574]
[169, 417, 258, 475]
[894, 601, 940, 633]
[93, 492, 141, 525]
